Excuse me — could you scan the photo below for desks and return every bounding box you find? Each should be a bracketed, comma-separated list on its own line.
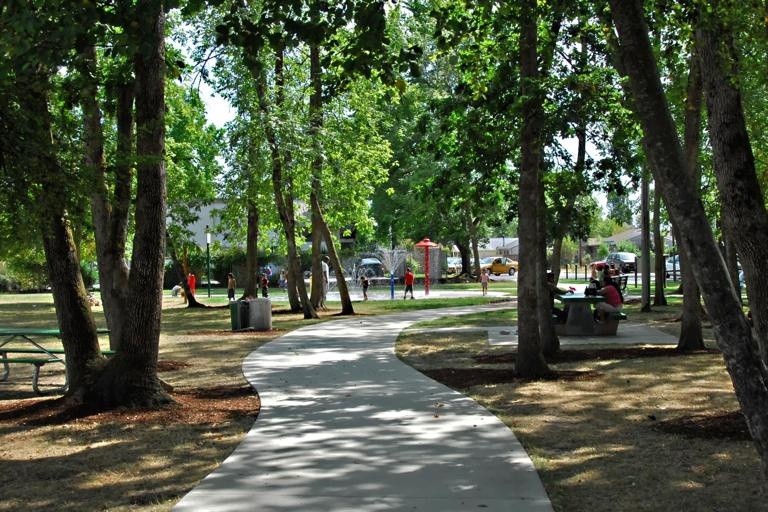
[0, 328, 111, 395]
[556, 292, 619, 336]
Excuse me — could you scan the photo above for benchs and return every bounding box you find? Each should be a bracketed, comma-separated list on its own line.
[0, 347, 117, 395]
[603, 312, 627, 335]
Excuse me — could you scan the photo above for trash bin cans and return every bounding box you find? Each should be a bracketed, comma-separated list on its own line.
[247, 298, 273, 332]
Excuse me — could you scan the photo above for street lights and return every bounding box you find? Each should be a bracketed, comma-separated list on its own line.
[204, 224, 212, 297]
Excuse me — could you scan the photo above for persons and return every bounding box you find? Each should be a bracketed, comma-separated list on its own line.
[182, 275, 188, 304]
[255, 268, 311, 300]
[171, 281, 184, 298]
[403, 268, 415, 301]
[738, 271, 747, 291]
[478, 269, 490, 297]
[359, 277, 371, 302]
[186, 272, 195, 297]
[321, 256, 331, 311]
[226, 273, 236, 305]
[545, 262, 624, 325]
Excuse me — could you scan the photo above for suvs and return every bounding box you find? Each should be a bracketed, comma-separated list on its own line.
[606, 252, 636, 273]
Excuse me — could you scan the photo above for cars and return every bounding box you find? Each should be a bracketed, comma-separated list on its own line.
[352, 257, 384, 280]
[589, 257, 609, 271]
[666, 255, 681, 278]
[446, 255, 519, 276]
